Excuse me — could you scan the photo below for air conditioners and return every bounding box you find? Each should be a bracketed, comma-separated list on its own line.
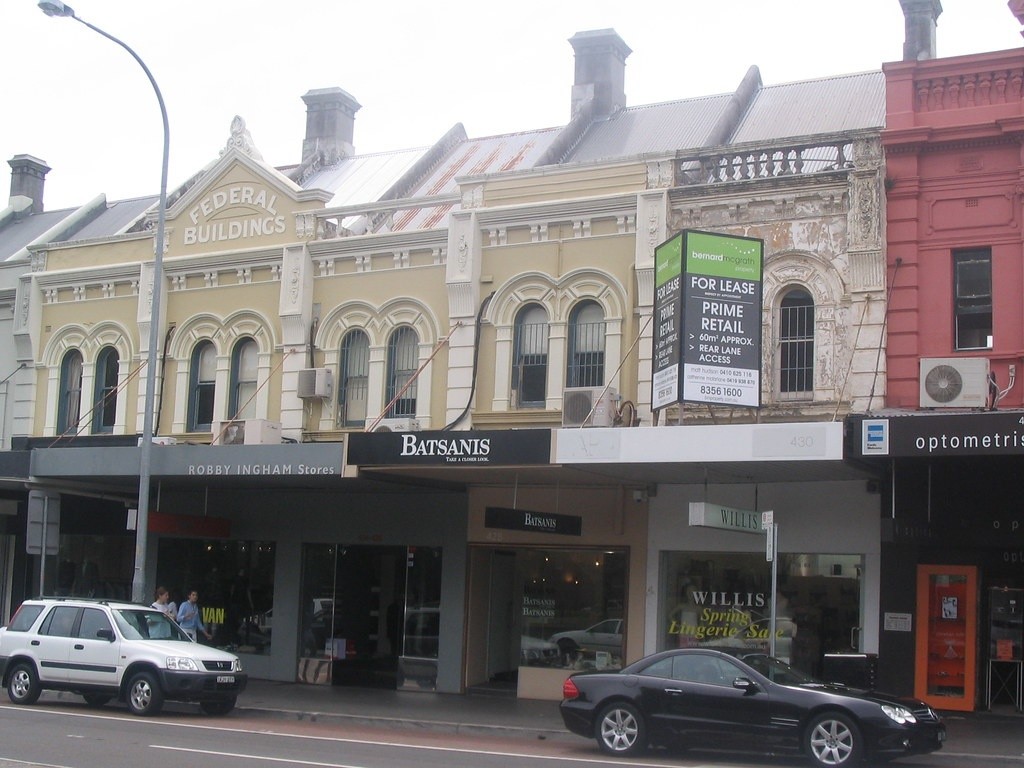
[560, 387, 622, 427]
[214, 420, 282, 445]
[138, 436, 177, 447]
[296, 368, 334, 401]
[919, 357, 991, 410]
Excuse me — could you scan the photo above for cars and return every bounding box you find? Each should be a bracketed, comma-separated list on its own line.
[559, 645, 948, 768]
[521, 633, 560, 670]
[548, 615, 627, 662]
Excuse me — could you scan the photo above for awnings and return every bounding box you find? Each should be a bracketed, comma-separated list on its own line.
[28, 407, 1024, 477]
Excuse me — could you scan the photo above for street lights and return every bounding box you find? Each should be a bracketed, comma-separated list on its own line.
[35, 1, 174, 604]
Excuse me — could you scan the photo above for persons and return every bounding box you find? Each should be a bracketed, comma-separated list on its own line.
[178, 592, 212, 643]
[144, 585, 177, 639]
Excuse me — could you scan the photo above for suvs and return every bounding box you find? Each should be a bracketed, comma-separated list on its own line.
[0, 598, 245, 715]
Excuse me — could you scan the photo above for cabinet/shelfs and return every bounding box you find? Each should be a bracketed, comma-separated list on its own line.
[914, 565, 978, 712]
